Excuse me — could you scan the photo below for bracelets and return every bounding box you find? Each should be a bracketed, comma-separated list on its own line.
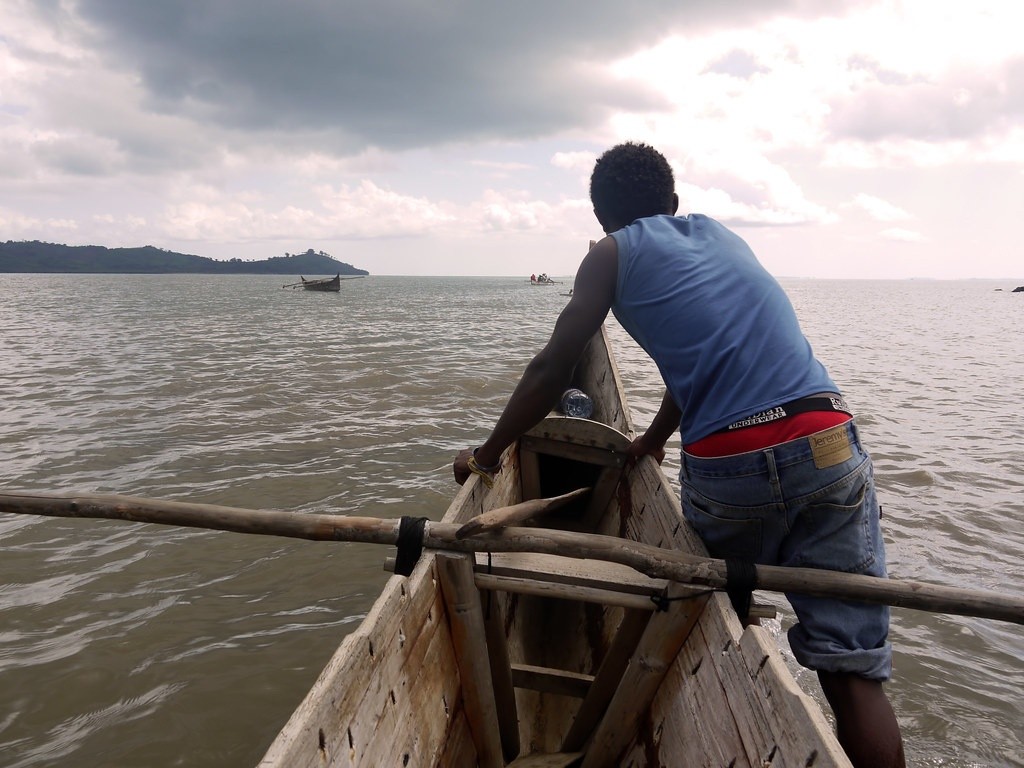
[468, 447, 501, 489]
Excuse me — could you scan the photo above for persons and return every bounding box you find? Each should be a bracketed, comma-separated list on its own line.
[531, 273, 547, 282]
[452, 142, 906, 768]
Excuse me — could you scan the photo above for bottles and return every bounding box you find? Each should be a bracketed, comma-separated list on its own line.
[561, 388, 593, 420]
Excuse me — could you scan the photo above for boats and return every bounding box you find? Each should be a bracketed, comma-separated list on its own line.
[300, 275, 341, 292]
[531, 279, 554, 286]
